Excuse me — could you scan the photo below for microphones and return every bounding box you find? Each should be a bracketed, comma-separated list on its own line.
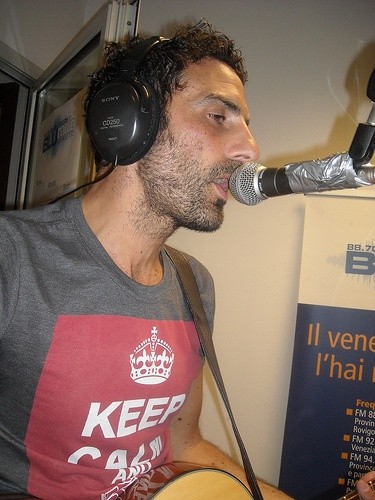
[228, 153, 375, 206]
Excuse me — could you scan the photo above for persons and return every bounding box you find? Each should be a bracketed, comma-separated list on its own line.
[0, 24, 375, 500]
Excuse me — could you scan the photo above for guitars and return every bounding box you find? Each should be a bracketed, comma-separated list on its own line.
[122, 461, 375, 500]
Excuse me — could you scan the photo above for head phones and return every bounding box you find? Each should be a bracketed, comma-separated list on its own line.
[86, 36, 170, 166]
